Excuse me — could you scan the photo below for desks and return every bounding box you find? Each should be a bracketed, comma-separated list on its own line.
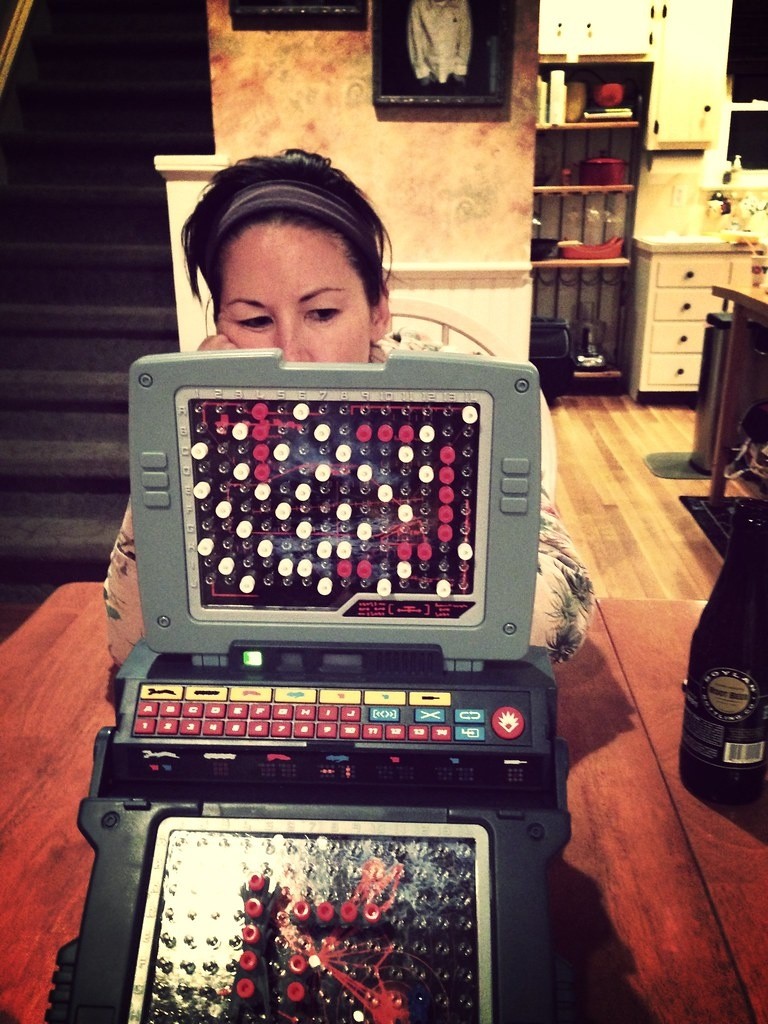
[0, 583, 768, 1024]
[707, 285, 768, 506]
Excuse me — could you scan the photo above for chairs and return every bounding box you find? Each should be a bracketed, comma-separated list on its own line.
[388, 295, 557, 504]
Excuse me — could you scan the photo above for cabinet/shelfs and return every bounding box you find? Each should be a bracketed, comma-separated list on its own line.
[626, 235, 768, 402]
[531, 53, 647, 391]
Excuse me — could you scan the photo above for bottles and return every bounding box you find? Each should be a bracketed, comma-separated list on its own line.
[680, 497, 768, 806]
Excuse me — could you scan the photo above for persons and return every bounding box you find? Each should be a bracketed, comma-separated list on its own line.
[407, 0, 472, 97]
[101, 147, 597, 670]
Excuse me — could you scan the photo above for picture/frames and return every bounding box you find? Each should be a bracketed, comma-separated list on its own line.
[226, 0, 370, 19]
[371, 0, 514, 107]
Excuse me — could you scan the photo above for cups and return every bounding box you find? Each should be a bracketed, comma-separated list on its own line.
[751, 256, 768, 287]
